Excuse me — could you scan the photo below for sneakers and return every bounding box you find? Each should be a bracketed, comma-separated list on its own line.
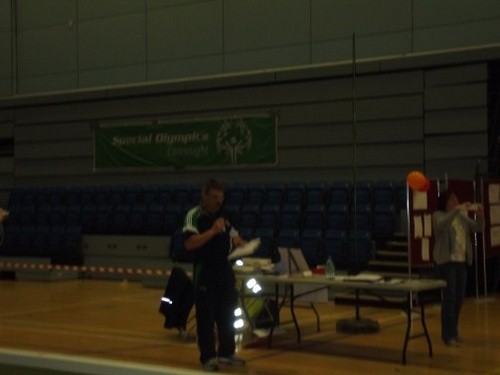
[215, 356, 246, 369]
[201, 358, 232, 374]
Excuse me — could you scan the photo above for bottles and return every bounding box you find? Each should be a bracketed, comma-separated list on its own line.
[327, 258, 334, 280]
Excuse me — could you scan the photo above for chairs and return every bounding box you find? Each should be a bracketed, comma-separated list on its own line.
[158, 268, 218, 340]
[1, 179, 412, 267]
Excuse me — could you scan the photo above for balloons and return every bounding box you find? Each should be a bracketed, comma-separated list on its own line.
[407, 171, 429, 189]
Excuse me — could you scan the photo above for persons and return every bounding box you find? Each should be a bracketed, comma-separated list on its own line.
[432, 191, 485, 348]
[182, 179, 247, 371]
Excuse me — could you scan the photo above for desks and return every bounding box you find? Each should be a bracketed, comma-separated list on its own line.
[235, 273, 448, 365]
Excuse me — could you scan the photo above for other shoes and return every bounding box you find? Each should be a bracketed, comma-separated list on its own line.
[445, 338, 462, 347]
